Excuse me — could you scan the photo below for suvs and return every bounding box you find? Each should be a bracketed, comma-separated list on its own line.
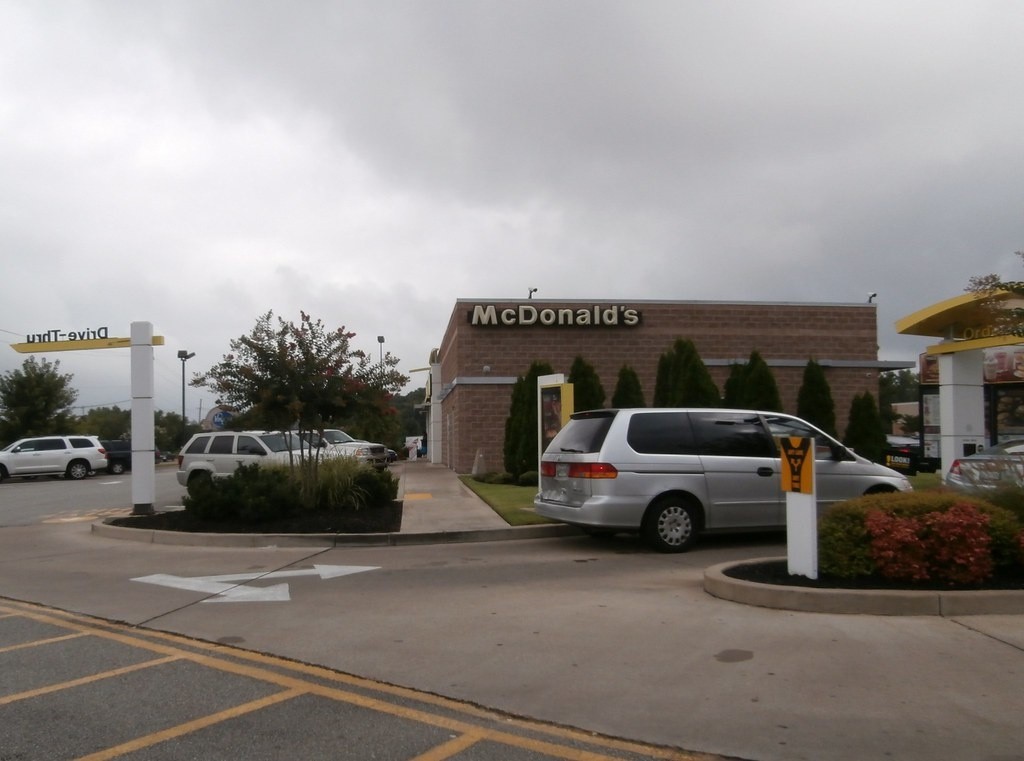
[176, 429, 397, 500]
[99, 439, 133, 476]
[534, 407, 914, 555]
[0, 434, 108, 483]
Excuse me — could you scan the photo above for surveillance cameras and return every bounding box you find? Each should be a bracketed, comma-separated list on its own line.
[528, 288, 537, 292]
[867, 293, 876, 297]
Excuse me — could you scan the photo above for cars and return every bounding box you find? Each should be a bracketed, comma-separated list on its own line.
[947, 437, 1024, 516]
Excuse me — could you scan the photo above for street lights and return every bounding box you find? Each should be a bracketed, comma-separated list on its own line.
[377, 335, 386, 372]
[178, 350, 196, 447]
